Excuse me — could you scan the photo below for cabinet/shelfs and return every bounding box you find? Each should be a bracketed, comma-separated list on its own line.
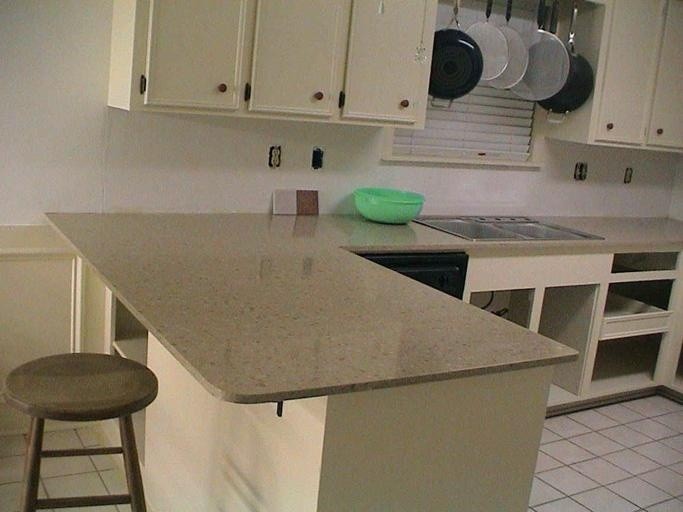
[249, 1, 341, 127]
[543, 0, 683, 155]
[108, 2, 247, 116]
[461, 246, 683, 418]
[339, 1, 435, 126]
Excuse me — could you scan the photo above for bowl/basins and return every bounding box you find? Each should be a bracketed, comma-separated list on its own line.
[352, 187, 425, 225]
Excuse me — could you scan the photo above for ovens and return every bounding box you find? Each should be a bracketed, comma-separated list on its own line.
[358, 251, 470, 302]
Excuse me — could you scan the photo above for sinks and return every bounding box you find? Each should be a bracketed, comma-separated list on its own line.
[408, 215, 527, 243]
[487, 214, 607, 243]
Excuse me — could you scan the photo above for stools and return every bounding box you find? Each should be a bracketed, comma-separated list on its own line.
[4, 352, 157, 512]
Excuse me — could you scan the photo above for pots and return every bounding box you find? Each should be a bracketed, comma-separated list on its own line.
[524, 1, 593, 124]
[428, 0, 484, 109]
